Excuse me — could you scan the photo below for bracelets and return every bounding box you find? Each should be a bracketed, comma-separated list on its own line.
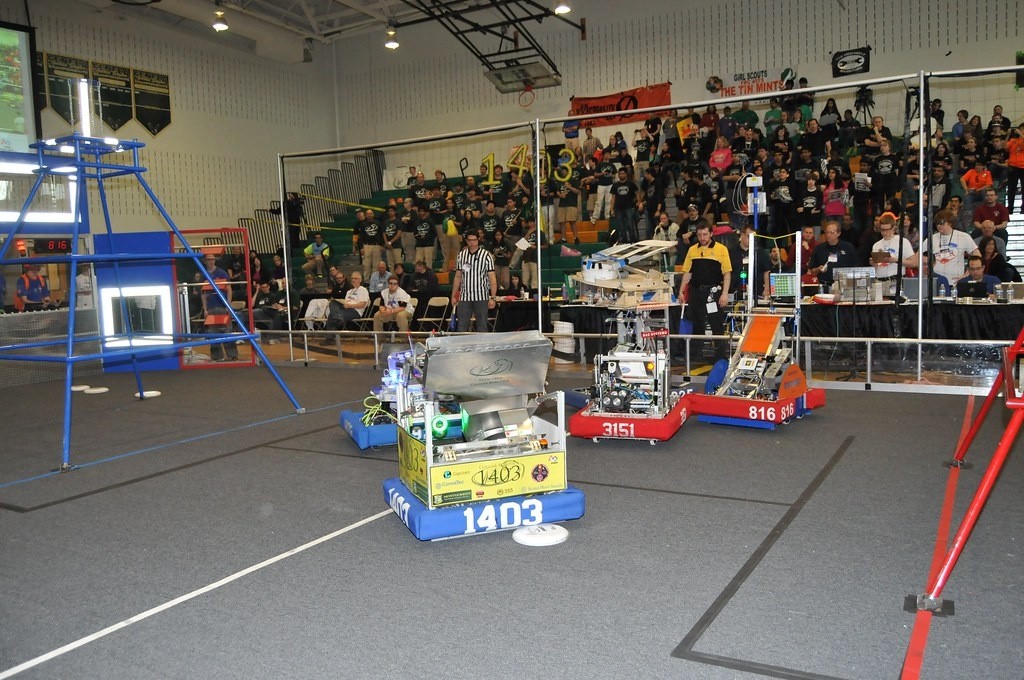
[390, 309, 394, 315]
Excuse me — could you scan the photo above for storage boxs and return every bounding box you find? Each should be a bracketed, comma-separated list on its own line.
[423, 330, 553, 400]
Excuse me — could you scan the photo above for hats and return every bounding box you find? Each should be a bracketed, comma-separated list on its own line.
[992, 121, 1004, 126]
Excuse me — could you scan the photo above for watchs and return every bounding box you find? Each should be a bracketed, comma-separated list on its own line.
[491, 296, 496, 301]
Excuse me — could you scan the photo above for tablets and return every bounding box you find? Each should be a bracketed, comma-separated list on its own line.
[872, 252, 891, 263]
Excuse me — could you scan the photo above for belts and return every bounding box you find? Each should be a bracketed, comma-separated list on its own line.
[876, 275, 897, 282]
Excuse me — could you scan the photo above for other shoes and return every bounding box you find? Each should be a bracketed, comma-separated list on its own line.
[555, 239, 567, 244]
[268, 339, 280, 345]
[575, 238, 579, 244]
[328, 321, 343, 329]
[590, 217, 596, 224]
[605, 215, 610, 220]
[320, 340, 335, 346]
[235, 340, 245, 344]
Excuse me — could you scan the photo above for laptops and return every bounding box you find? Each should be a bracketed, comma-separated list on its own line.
[1001, 282, 1024, 299]
[957, 281, 987, 298]
[902, 278, 937, 301]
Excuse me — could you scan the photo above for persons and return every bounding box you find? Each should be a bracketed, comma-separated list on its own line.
[13, 263, 50, 312]
[76, 263, 93, 308]
[186, 77, 1024, 364]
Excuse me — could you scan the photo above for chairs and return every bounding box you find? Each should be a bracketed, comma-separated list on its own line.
[190, 300, 289, 333]
[548, 286, 562, 297]
[299, 298, 501, 344]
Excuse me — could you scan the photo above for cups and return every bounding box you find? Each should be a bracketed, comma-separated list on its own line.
[873, 284, 882, 301]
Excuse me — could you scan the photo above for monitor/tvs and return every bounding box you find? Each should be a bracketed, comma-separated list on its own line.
[769, 272, 796, 307]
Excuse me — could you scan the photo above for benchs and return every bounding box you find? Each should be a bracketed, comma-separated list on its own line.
[189, 151, 1006, 297]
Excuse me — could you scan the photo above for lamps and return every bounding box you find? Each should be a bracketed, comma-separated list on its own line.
[213, 6, 228, 32]
[384, 26, 399, 49]
[555, 0, 571, 15]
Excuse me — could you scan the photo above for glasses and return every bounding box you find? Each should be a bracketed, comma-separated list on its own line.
[969, 266, 983, 272]
[824, 231, 836, 235]
[467, 238, 477, 241]
[935, 220, 945, 225]
[205, 259, 215, 261]
[879, 226, 893, 232]
[389, 282, 398, 285]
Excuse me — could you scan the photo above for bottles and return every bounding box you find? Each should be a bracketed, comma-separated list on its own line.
[940, 284, 945, 298]
[520, 287, 524, 297]
[562, 284, 567, 299]
[951, 284, 957, 300]
[635, 285, 643, 303]
[995, 285, 1003, 298]
[819, 285, 824, 294]
[587, 290, 593, 305]
[1007, 285, 1014, 301]
[740, 267, 747, 292]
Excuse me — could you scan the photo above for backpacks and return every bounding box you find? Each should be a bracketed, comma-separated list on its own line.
[14, 272, 45, 311]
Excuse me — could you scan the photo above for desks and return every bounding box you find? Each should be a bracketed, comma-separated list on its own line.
[752, 298, 1024, 375]
[295, 294, 555, 338]
[559, 305, 693, 368]
